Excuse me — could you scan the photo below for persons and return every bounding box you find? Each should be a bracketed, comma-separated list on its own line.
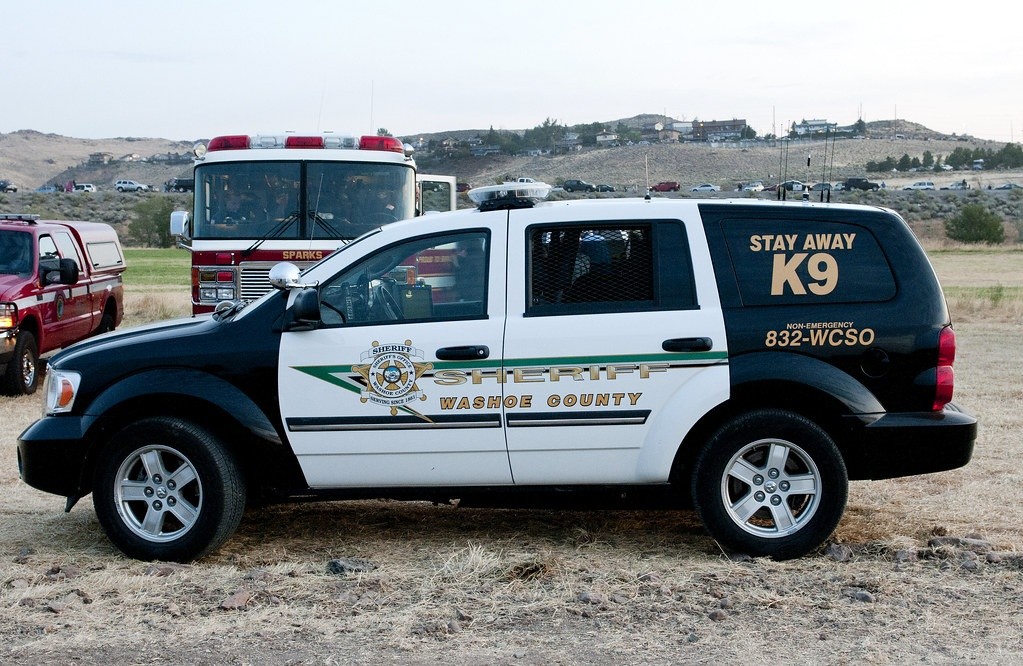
[506, 174, 517, 183]
[161, 180, 189, 194]
[961, 179, 969, 189]
[212, 190, 249, 224]
[52, 181, 72, 192]
[880, 181, 886, 188]
[737, 181, 743, 191]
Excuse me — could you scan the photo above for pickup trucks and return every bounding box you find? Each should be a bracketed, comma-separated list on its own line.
[173, 176, 194, 194]
[0, 213, 124, 397]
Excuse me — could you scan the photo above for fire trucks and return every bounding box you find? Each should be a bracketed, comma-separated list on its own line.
[169, 129, 474, 320]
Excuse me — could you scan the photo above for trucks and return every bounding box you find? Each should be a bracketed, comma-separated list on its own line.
[501, 177, 536, 185]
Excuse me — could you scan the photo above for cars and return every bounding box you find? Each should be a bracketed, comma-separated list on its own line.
[456, 182, 473, 191]
[689, 183, 722, 193]
[995, 184, 1023, 190]
[834, 182, 843, 191]
[423, 181, 443, 193]
[734, 181, 778, 192]
[75, 184, 96, 193]
[940, 182, 970, 191]
[775, 180, 813, 191]
[553, 185, 564, 189]
[5, 184, 19, 193]
[596, 185, 617, 192]
[31, 186, 56, 193]
[809, 183, 833, 192]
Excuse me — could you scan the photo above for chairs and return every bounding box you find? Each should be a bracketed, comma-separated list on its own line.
[541, 229, 582, 303]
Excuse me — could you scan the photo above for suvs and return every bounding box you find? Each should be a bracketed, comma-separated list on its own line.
[649, 181, 680, 193]
[115, 180, 160, 193]
[562, 179, 598, 194]
[901, 182, 936, 190]
[11, 177, 982, 558]
[842, 176, 881, 191]
